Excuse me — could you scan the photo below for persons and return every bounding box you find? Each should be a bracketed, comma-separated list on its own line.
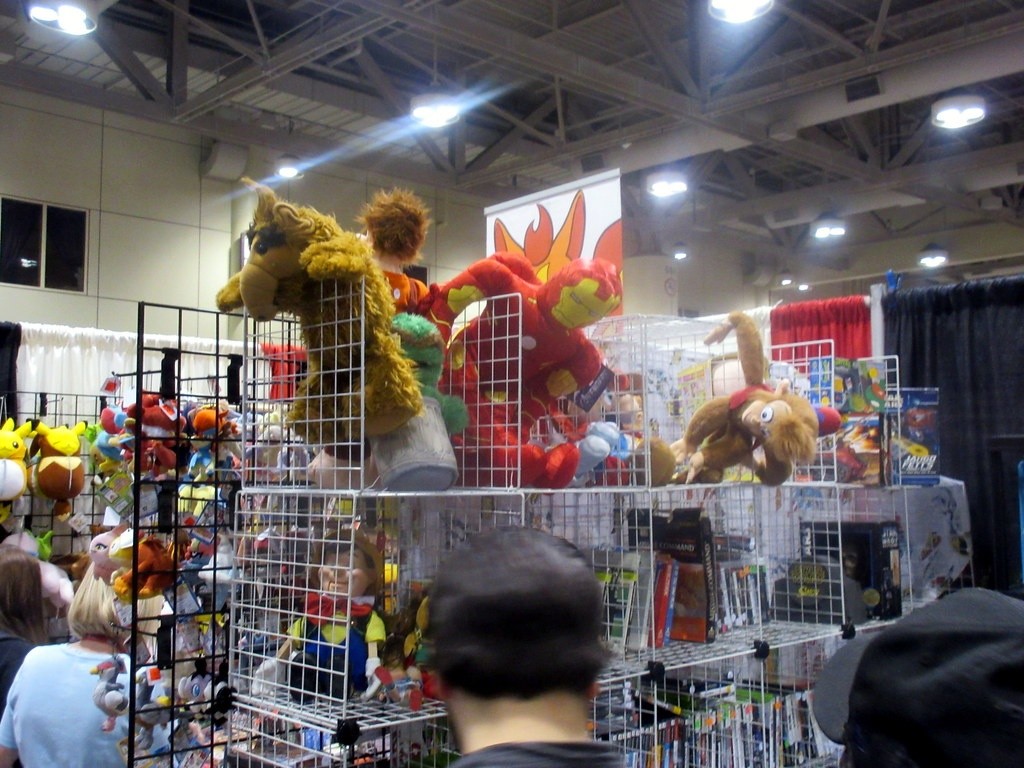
[0, 559, 180, 768]
[839, 575, 1024, 768]
[601, 574, 617, 638]
[0, 546, 47, 711]
[429, 527, 623, 768]
[252, 530, 386, 704]
[361, 186, 429, 314]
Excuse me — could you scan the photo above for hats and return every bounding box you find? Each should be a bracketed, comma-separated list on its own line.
[811, 588, 1024, 768]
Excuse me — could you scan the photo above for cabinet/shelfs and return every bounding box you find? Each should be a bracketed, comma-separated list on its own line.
[225, 267, 913, 768]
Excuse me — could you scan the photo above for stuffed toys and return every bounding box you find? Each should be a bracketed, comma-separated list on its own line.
[0, 174, 842, 750]
